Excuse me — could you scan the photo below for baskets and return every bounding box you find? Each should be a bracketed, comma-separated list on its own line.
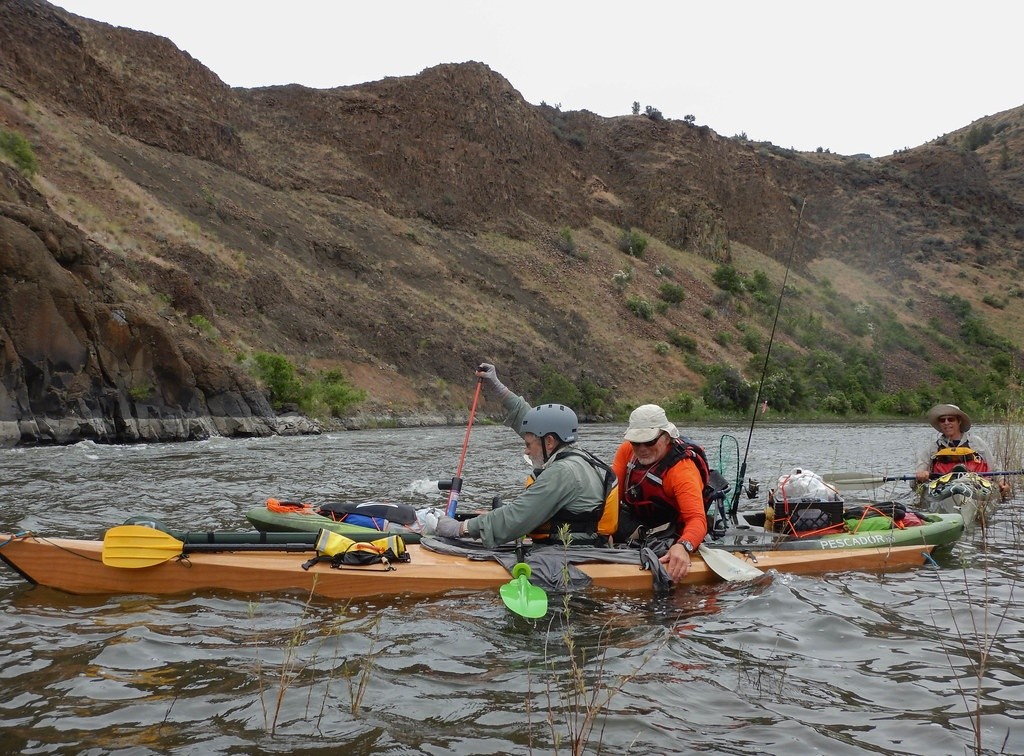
[769, 488, 843, 533]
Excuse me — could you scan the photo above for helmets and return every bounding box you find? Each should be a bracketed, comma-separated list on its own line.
[518, 403, 578, 443]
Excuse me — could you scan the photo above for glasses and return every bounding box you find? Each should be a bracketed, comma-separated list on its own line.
[630, 431, 666, 447]
[937, 418, 958, 422]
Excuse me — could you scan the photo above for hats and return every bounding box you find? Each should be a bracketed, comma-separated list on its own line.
[625, 404, 680, 444]
[928, 403, 971, 433]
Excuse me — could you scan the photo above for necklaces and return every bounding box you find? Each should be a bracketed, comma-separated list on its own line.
[624, 452, 661, 498]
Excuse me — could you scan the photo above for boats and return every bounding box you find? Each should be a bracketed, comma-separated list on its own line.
[1, 511, 968, 604]
[916, 478, 1003, 528]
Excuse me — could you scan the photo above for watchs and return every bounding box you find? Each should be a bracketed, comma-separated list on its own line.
[677, 539, 695, 554]
[462, 518, 471, 538]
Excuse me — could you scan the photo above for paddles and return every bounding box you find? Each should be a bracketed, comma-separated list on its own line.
[822, 468, 1023, 492]
[445, 376, 485, 529]
[495, 531, 551, 619]
[102, 525, 321, 570]
[671, 526, 766, 583]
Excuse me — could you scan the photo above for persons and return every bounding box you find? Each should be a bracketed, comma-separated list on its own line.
[914, 403, 1011, 497]
[610, 402, 711, 585]
[434, 361, 605, 550]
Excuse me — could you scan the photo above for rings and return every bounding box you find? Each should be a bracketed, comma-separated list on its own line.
[1005, 491, 1008, 494]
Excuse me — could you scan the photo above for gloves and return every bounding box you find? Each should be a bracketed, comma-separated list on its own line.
[435, 516, 461, 538]
